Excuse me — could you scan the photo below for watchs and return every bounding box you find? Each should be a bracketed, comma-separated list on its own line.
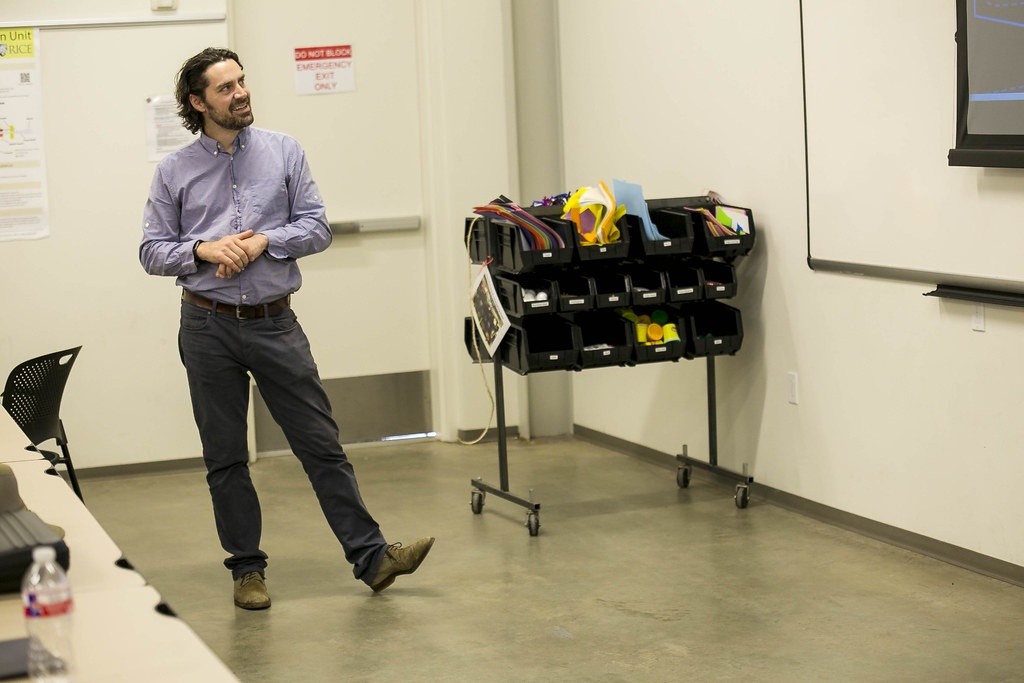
[193, 241, 206, 266]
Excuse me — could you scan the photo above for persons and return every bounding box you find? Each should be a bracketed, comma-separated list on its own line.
[138, 47, 436, 611]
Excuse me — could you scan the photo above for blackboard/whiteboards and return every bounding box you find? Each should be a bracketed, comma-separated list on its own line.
[797, 0, 1023, 308]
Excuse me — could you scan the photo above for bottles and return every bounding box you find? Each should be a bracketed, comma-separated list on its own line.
[22, 546, 74, 683]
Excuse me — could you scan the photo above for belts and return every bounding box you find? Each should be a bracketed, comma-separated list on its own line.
[181, 289, 290, 319]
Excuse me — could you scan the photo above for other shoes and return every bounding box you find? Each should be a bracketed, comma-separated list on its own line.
[233, 571, 271, 608]
[370, 537, 435, 592]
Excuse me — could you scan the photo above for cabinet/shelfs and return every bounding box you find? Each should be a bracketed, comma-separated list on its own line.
[463, 192, 756, 537]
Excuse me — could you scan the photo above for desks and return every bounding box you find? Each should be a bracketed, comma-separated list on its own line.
[0, 404, 241, 683]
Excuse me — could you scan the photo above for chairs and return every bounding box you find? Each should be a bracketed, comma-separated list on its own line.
[0, 345, 87, 507]
